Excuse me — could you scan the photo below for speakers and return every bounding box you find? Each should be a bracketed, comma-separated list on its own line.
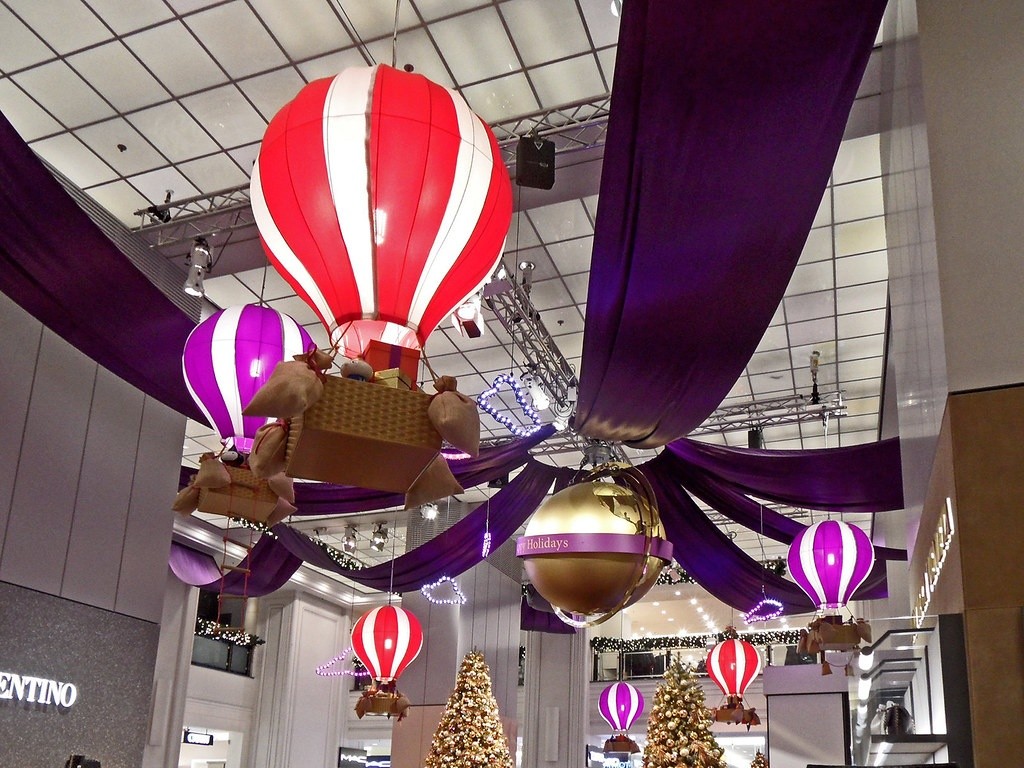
[516, 136, 555, 190]
[748, 430, 763, 448]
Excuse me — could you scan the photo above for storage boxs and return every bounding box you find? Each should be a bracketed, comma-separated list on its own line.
[606, 742, 632, 752]
[715, 709, 749, 724]
[815, 626, 862, 652]
[196, 465, 281, 523]
[283, 373, 444, 494]
[364, 696, 401, 716]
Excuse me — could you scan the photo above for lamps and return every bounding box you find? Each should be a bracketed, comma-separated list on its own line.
[450, 287, 486, 340]
[597, 610, 644, 732]
[519, 371, 550, 411]
[348, 506, 424, 683]
[704, 607, 763, 699]
[180, 256, 318, 458]
[551, 399, 578, 431]
[184, 241, 214, 298]
[248, 0, 515, 365]
[785, 426, 875, 611]
